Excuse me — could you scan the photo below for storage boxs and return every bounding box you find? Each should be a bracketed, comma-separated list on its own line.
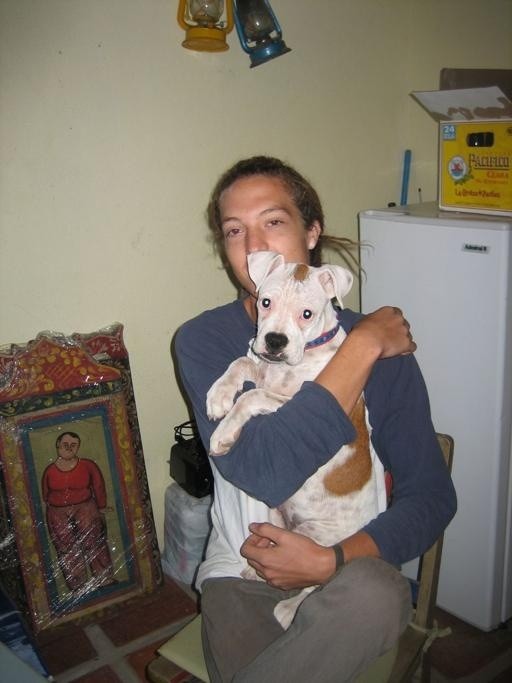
[412, 65, 512, 220]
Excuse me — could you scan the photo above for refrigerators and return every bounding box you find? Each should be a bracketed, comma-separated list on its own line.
[358, 198, 512, 634]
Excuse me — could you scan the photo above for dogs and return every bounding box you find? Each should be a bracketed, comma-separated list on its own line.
[205, 251, 378, 631]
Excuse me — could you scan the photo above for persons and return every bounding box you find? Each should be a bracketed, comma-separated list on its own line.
[174, 157, 457, 683]
[41, 432, 118, 596]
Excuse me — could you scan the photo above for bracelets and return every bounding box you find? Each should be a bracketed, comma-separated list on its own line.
[332, 544, 345, 572]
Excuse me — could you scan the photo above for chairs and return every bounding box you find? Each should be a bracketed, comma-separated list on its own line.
[146, 432, 456, 681]
